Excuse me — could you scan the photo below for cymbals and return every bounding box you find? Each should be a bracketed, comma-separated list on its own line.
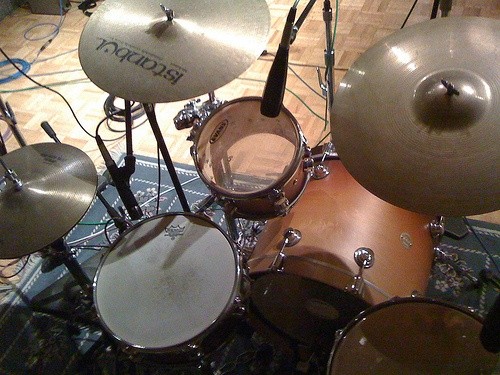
[78, 0, 271, 104]
[0, 142, 99, 260]
[329, 15, 500, 216]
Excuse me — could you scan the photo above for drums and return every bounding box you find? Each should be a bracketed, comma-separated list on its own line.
[90, 211, 255, 367]
[326, 294, 500, 375]
[240, 142, 446, 353]
[190, 95, 313, 221]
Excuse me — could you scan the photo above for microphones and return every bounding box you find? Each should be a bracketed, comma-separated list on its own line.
[95, 135, 144, 221]
[260, 7, 297, 118]
